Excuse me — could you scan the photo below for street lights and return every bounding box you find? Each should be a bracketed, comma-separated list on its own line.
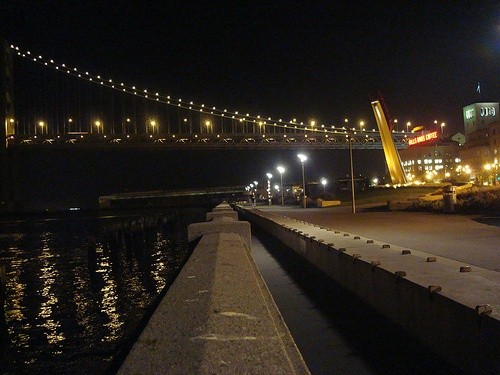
[297, 153, 307, 208]
[40, 121, 44, 134]
[311, 121, 315, 133]
[440, 122, 446, 134]
[344, 118, 350, 129]
[277, 166, 285, 206]
[393, 119, 399, 133]
[359, 120, 364, 130]
[265, 172, 273, 206]
[151, 120, 156, 134]
[406, 122, 411, 132]
[94, 120, 100, 134]
[205, 120, 211, 134]
[258, 122, 262, 133]
